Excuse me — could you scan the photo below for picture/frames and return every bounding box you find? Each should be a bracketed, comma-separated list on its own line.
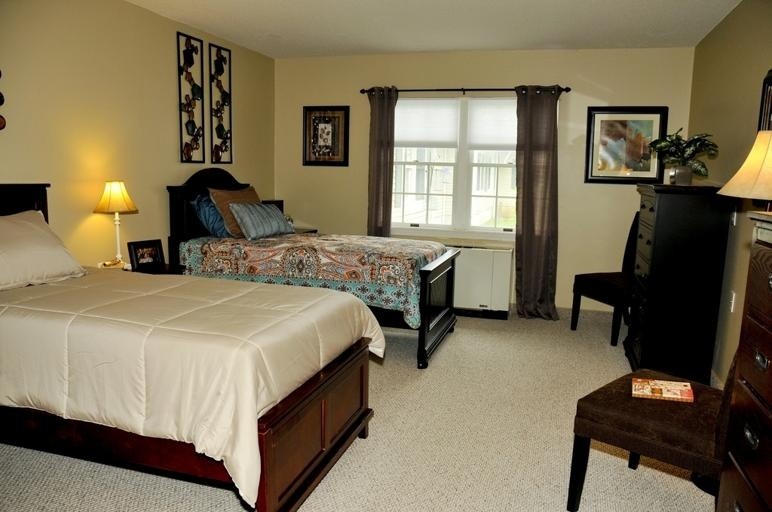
[127, 240, 166, 272]
[751, 68, 772, 208]
[176, 31, 206, 164]
[303, 106, 350, 167]
[208, 42, 233, 164]
[584, 106, 669, 185]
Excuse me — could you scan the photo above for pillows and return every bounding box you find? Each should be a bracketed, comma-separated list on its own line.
[191, 195, 231, 238]
[0, 210, 88, 291]
[208, 186, 261, 238]
[228, 202, 296, 241]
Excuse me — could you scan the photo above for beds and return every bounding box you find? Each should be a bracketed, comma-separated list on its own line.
[166, 168, 461, 369]
[0, 183, 386, 512]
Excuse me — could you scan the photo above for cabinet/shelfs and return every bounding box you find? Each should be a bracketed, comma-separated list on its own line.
[622, 185, 734, 386]
[715, 210, 772, 512]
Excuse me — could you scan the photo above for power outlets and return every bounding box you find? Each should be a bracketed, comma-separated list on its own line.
[726, 290, 735, 313]
[730, 212, 736, 226]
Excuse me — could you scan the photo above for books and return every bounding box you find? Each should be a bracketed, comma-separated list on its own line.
[632, 378, 693, 402]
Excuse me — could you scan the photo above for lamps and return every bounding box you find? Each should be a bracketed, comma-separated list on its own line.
[93, 181, 139, 269]
[717, 130, 772, 200]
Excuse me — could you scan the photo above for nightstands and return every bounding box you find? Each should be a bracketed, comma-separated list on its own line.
[294, 228, 318, 234]
[143, 264, 186, 275]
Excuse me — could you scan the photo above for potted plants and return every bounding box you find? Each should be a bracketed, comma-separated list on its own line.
[649, 127, 719, 186]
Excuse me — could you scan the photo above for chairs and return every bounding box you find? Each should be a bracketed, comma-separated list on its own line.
[571, 211, 640, 346]
[567, 344, 739, 511]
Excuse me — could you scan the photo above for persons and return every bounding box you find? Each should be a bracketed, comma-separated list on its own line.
[600, 120, 651, 172]
